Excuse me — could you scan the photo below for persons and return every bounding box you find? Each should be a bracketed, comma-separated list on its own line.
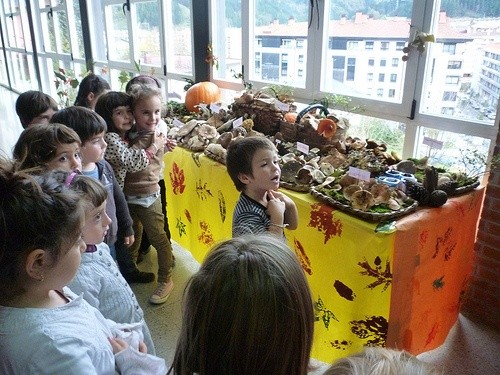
[162, 232, 316, 375]
[321, 346, 441, 375]
[124, 74, 176, 265]
[49, 106, 136, 265]
[92, 90, 168, 283]
[11, 124, 82, 182]
[0, 157, 167, 375]
[49, 168, 157, 358]
[15, 90, 59, 132]
[74, 73, 111, 111]
[225, 134, 298, 243]
[124, 87, 174, 304]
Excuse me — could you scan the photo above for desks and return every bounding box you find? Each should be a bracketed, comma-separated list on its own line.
[162, 130, 487, 363]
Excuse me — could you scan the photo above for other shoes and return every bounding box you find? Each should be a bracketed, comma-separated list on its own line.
[135, 254, 143, 264]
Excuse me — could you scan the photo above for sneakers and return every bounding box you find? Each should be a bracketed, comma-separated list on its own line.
[121, 270, 155, 283]
[149, 277, 174, 304]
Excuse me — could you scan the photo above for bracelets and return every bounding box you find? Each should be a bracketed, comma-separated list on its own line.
[270, 222, 290, 228]
[151, 141, 159, 153]
